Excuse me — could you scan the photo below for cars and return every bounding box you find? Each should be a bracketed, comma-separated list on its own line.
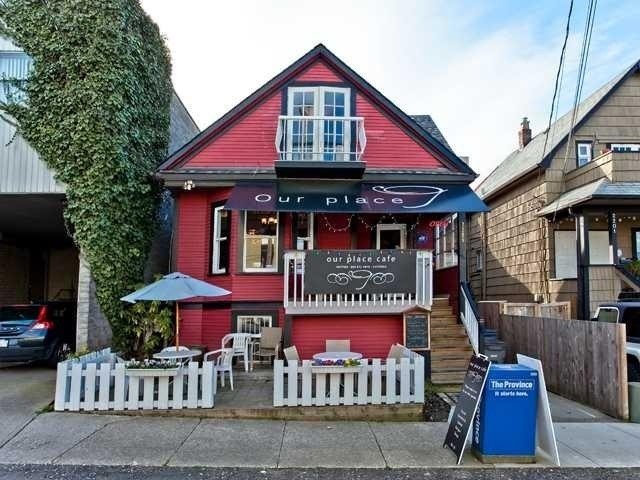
[0, 300, 77, 368]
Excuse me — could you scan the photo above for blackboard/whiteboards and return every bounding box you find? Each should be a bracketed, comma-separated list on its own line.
[403, 312, 430, 350]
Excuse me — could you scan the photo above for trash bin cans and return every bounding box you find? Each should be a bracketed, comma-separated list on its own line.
[628, 382, 640, 421]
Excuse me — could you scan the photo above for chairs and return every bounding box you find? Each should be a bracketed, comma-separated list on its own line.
[382, 344, 405, 394]
[326, 340, 350, 352]
[221, 333, 251, 372]
[251, 327, 283, 371]
[162, 346, 193, 366]
[203, 348, 235, 392]
[283, 345, 315, 395]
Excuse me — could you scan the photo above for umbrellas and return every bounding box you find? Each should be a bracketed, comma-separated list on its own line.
[118, 269, 236, 354]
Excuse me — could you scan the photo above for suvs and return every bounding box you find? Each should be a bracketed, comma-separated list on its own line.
[591, 288, 640, 383]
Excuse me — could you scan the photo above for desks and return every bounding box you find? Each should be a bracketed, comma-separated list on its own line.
[152, 350, 203, 394]
[312, 351, 363, 397]
